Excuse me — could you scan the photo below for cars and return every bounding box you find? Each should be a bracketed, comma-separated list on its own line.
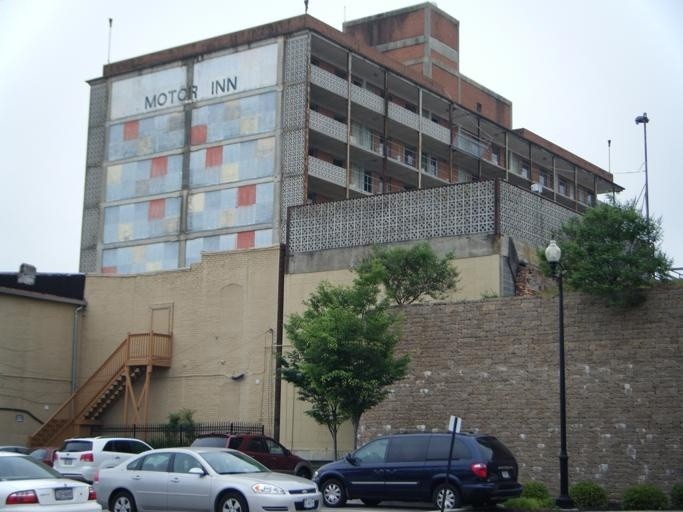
[311, 431, 523, 511]
[0, 445, 103, 512]
[189, 431, 316, 480]
[52, 436, 155, 484]
[93, 446, 324, 512]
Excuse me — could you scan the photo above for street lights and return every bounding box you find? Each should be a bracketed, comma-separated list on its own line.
[635, 112, 651, 279]
[545, 238, 574, 509]
